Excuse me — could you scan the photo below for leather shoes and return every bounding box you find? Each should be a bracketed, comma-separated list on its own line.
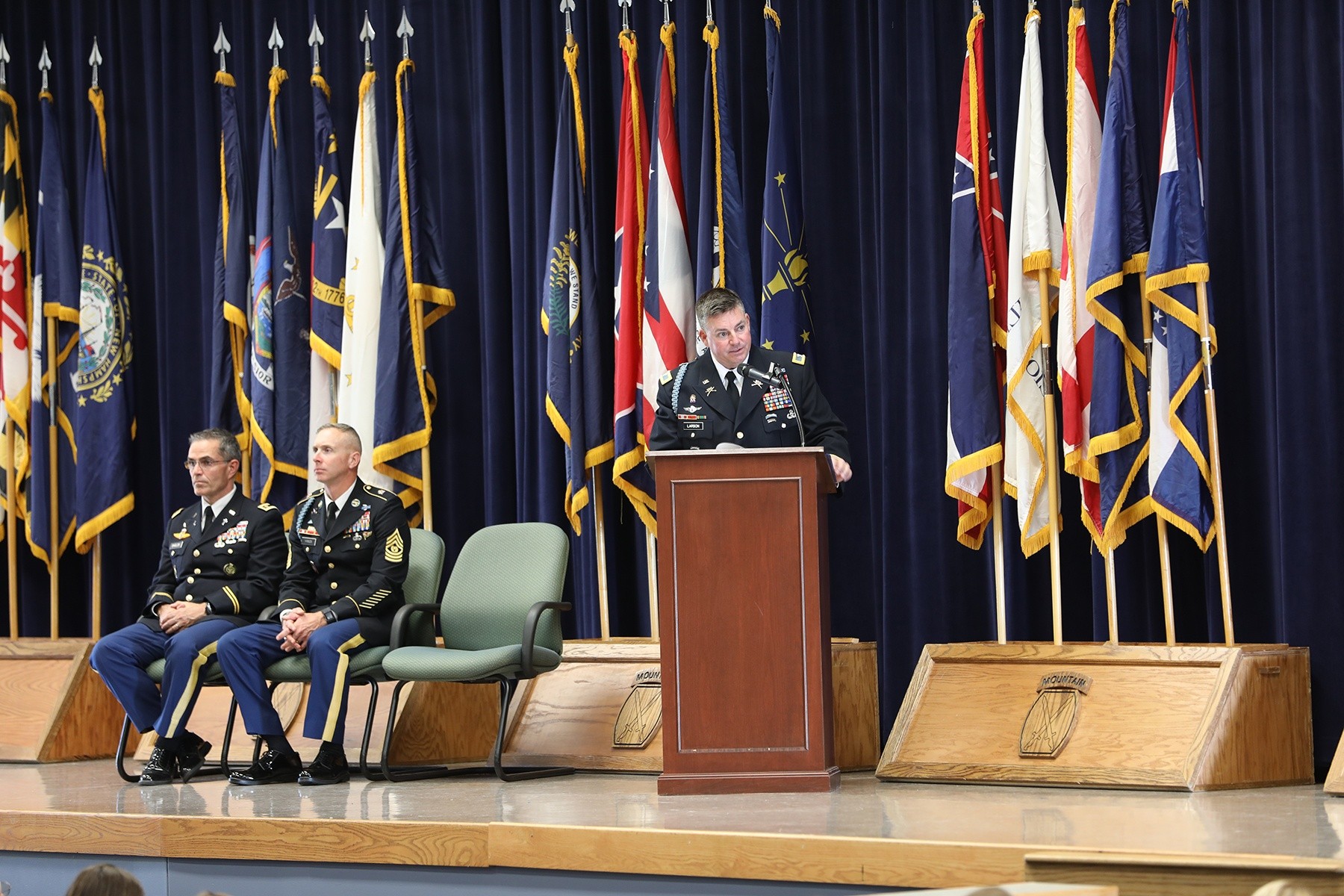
[229, 750, 303, 785]
[298, 750, 350, 784]
[138, 745, 174, 785]
[176, 733, 212, 783]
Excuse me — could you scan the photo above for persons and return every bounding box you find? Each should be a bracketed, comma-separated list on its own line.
[217, 423, 412, 785]
[89, 430, 289, 786]
[649, 288, 853, 484]
[63, 864, 143, 896]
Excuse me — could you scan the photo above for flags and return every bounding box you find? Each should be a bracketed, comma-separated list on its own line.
[0, 87, 138, 575]
[209, 58, 456, 532]
[944, 0, 1218, 559]
[540, 7, 819, 539]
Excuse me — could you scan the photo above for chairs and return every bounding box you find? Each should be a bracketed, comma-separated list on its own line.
[116, 521, 570, 786]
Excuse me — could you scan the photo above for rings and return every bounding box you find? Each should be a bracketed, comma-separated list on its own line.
[175, 623, 180, 629]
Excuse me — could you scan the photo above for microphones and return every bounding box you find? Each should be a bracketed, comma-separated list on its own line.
[737, 364, 783, 389]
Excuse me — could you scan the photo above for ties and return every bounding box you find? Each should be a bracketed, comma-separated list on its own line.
[325, 501, 339, 534]
[725, 371, 740, 406]
[200, 507, 214, 535]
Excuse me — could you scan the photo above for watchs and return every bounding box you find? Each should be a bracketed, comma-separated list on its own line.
[205, 602, 214, 615]
[321, 607, 337, 624]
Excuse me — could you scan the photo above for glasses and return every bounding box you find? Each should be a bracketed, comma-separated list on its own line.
[184, 459, 232, 470]
